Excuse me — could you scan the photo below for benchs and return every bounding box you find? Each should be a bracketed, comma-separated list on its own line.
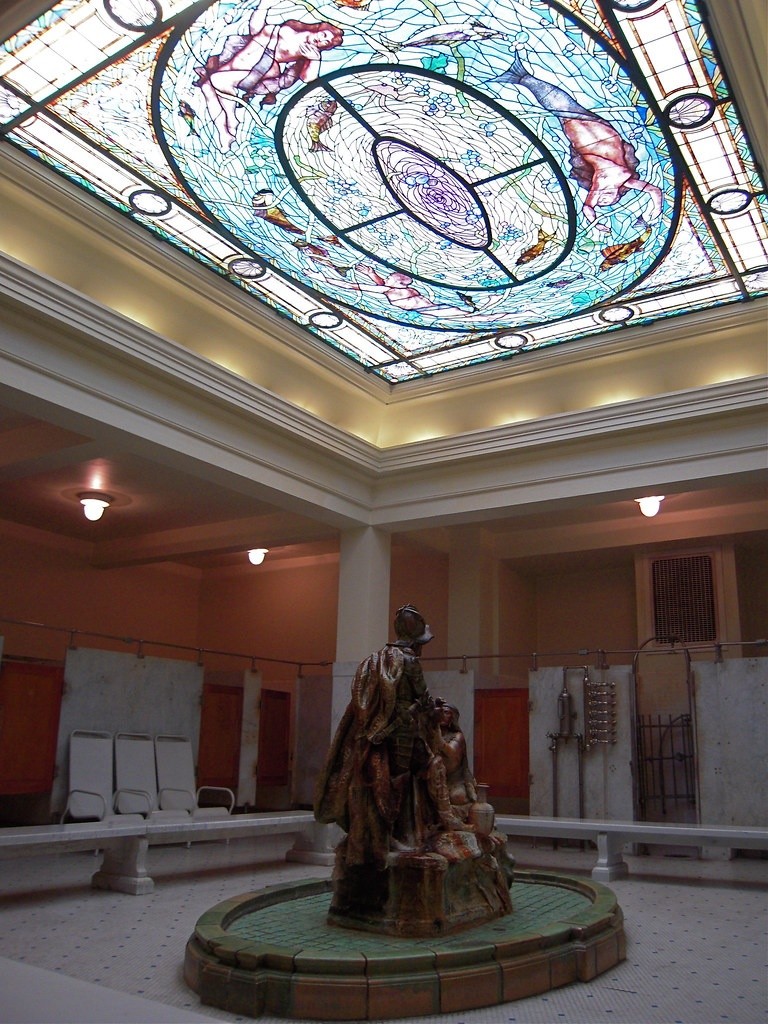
[0, 809, 341, 897]
[495, 814, 768, 882]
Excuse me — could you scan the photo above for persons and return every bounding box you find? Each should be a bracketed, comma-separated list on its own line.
[314, 604, 477, 871]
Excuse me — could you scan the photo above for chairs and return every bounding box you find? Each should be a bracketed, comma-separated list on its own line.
[155, 733, 235, 850]
[113, 731, 194, 849]
[59, 729, 152, 859]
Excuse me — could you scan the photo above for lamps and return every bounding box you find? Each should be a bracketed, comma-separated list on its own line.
[633, 496, 666, 519]
[246, 548, 269, 566]
[77, 493, 113, 522]
